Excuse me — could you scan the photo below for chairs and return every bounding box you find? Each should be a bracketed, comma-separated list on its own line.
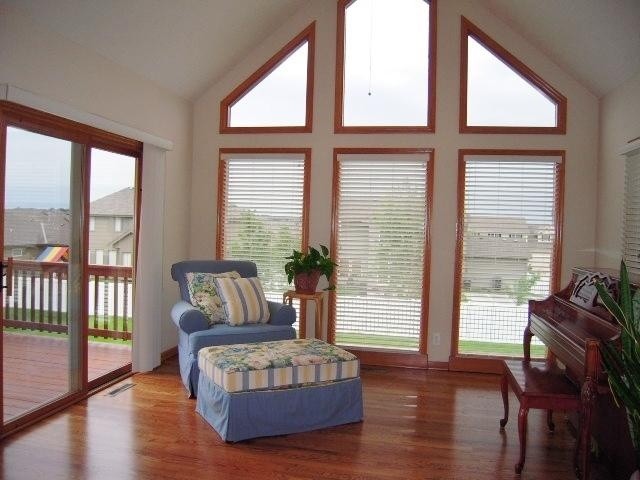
[171, 260, 296, 397]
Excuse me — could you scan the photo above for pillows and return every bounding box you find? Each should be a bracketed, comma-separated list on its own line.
[183, 271, 271, 326]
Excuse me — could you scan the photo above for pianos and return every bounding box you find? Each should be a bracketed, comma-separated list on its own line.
[522, 267, 640, 480]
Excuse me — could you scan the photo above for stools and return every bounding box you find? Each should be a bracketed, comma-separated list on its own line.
[196, 337, 363, 442]
[500, 359, 583, 480]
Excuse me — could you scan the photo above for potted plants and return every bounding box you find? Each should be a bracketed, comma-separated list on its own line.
[284, 243, 338, 294]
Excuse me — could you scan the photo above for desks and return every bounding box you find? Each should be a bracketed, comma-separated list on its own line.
[283, 290, 324, 340]
